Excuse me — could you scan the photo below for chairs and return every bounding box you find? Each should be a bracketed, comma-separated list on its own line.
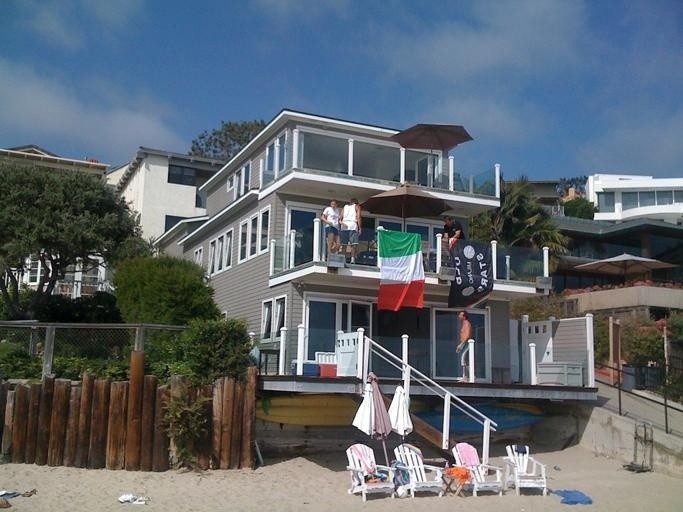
[345, 444, 397, 503]
[505, 444, 547, 496]
[394, 443, 445, 499]
[451, 442, 504, 497]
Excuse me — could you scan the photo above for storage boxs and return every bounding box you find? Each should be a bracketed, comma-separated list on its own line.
[291, 359, 319, 376]
[319, 363, 337, 377]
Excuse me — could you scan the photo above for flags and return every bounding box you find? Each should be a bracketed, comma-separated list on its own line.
[448, 238, 493, 310]
[377, 228, 426, 311]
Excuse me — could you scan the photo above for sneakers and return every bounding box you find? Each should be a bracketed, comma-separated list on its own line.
[457, 377, 467, 382]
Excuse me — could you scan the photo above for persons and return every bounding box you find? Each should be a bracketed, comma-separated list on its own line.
[441, 215, 466, 240]
[338, 197, 362, 265]
[36, 342, 44, 356]
[455, 310, 472, 382]
[320, 199, 341, 254]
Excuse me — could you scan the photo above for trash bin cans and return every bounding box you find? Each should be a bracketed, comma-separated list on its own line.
[623, 363, 662, 390]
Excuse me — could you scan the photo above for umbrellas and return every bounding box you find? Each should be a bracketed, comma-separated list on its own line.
[372, 376, 393, 467]
[389, 120, 474, 188]
[388, 385, 414, 443]
[358, 183, 454, 232]
[351, 380, 375, 440]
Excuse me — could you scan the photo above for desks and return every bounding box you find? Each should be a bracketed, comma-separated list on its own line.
[442, 467, 471, 498]
